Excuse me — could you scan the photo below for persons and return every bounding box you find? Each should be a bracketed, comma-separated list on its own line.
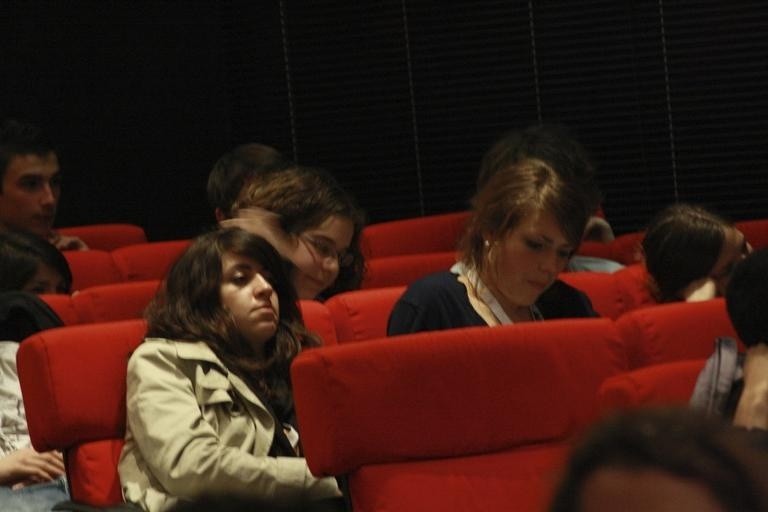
[0, 221, 73, 293]
[207, 144, 280, 218]
[643, 204, 752, 301]
[385, 157, 601, 336]
[116, 227, 340, 511]
[724, 245, 767, 429]
[221, 168, 368, 301]
[0, 342, 67, 512]
[1, 134, 87, 251]
[547, 406, 765, 511]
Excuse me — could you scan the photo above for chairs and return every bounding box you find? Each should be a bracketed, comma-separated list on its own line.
[16, 208, 768, 512]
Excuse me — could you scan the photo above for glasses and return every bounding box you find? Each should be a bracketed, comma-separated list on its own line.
[303, 232, 353, 267]
